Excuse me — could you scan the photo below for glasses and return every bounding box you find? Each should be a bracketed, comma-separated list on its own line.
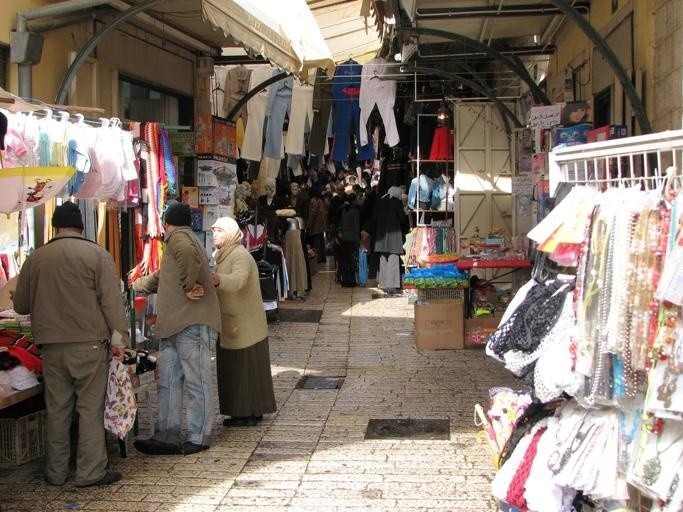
[436, 82, 451, 121]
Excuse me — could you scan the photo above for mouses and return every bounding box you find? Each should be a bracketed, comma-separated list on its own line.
[15, 106, 123, 132]
[212, 50, 384, 100]
[255, 253, 273, 271]
[614, 175, 666, 196]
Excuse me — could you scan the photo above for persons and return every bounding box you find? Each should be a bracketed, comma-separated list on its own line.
[128, 203, 222, 455]
[210, 216, 276, 427]
[304, 171, 369, 288]
[13, 200, 130, 486]
[235, 181, 309, 224]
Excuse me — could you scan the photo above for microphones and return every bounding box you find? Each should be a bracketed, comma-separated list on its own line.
[105, 385, 156, 461]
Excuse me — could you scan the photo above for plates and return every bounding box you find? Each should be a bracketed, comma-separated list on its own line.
[406, 158, 454, 215]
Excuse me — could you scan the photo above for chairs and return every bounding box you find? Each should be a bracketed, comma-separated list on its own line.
[223, 414, 263, 426]
[91, 472, 122, 485]
[133, 438, 209, 455]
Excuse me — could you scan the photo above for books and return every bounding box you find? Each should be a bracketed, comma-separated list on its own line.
[413, 286, 506, 352]
[169, 115, 239, 260]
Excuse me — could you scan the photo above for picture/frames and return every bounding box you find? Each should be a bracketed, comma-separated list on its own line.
[457, 257, 533, 303]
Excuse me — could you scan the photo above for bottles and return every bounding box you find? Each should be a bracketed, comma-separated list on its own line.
[164, 202, 191, 226]
[51, 201, 84, 229]
